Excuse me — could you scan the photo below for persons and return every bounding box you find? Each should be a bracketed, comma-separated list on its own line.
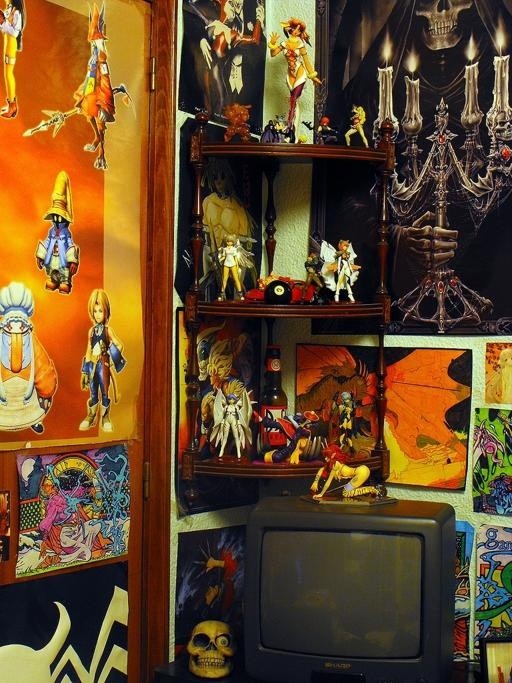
[217, 234, 255, 300]
[200, 13, 382, 499]
[210, 381, 381, 498]
[268, 18, 322, 143]
[303, 239, 361, 303]
[314, 117, 340, 145]
[262, 118, 284, 143]
[345, 106, 370, 148]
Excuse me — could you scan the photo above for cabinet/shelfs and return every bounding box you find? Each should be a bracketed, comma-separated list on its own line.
[182, 112, 396, 481]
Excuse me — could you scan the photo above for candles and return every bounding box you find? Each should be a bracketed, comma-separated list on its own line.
[378, 15, 512, 122]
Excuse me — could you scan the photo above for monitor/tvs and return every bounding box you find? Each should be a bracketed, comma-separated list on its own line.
[243, 496, 456, 683]
